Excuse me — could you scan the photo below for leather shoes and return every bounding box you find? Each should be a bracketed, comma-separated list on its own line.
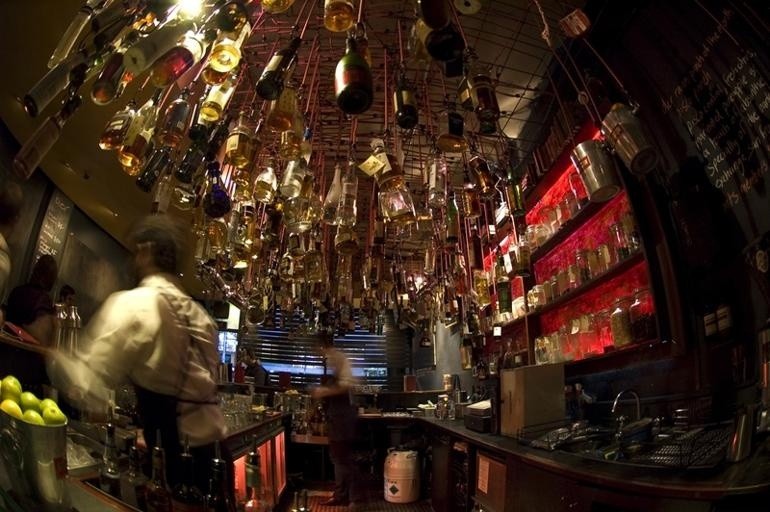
[319, 498, 349, 506]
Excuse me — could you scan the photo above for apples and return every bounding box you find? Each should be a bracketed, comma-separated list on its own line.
[0, 375, 66, 425]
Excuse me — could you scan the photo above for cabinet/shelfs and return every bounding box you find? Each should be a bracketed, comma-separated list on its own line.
[476, 44, 702, 428]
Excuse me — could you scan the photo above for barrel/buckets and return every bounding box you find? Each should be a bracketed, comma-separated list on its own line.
[383, 444, 422, 502]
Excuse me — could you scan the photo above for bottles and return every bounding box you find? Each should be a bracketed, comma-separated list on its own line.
[14, 0, 662, 512]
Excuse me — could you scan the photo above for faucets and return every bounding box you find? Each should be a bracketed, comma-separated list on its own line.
[611, 388, 641, 417]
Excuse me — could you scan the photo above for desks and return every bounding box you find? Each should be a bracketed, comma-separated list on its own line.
[206, 407, 298, 511]
[354, 408, 770, 511]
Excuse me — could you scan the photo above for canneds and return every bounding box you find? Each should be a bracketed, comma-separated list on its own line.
[534, 286, 657, 367]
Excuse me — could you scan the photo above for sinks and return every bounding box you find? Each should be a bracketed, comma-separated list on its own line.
[563, 432, 615, 458]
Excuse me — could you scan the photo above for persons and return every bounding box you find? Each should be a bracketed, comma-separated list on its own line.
[58, 284, 75, 307]
[45, 226, 236, 511]
[8, 255, 59, 327]
[310, 330, 370, 511]
[242, 346, 272, 385]
